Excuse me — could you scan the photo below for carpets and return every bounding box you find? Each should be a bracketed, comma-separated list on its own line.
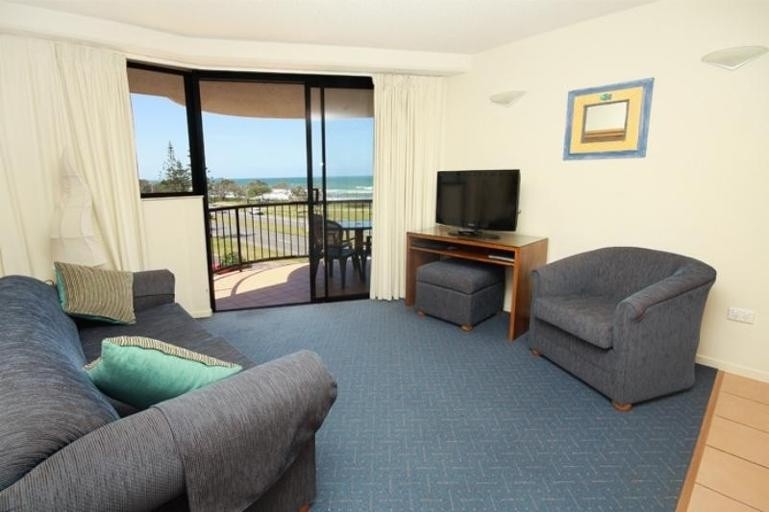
[194, 299, 718, 512]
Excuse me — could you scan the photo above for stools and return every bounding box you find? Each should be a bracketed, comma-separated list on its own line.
[415, 259, 505, 330]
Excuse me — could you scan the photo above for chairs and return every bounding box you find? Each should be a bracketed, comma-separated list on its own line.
[310, 212, 373, 289]
[529, 246, 717, 411]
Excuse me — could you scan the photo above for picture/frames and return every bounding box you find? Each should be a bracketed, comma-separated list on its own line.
[561, 76, 655, 160]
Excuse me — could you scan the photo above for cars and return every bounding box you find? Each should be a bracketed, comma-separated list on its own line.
[249, 208, 261, 215]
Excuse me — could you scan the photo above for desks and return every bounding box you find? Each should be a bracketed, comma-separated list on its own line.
[405, 224, 547, 342]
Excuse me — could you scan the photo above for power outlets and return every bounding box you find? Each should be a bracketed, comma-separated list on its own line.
[727, 307, 755, 325]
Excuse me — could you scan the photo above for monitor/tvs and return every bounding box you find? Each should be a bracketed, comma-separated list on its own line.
[436, 169, 520, 240]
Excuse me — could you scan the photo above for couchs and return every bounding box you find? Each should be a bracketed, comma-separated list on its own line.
[0, 268, 337, 512]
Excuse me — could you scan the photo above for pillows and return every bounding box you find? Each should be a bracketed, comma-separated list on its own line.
[51, 260, 243, 412]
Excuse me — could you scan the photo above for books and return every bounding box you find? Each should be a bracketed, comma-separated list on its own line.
[486, 249, 515, 263]
